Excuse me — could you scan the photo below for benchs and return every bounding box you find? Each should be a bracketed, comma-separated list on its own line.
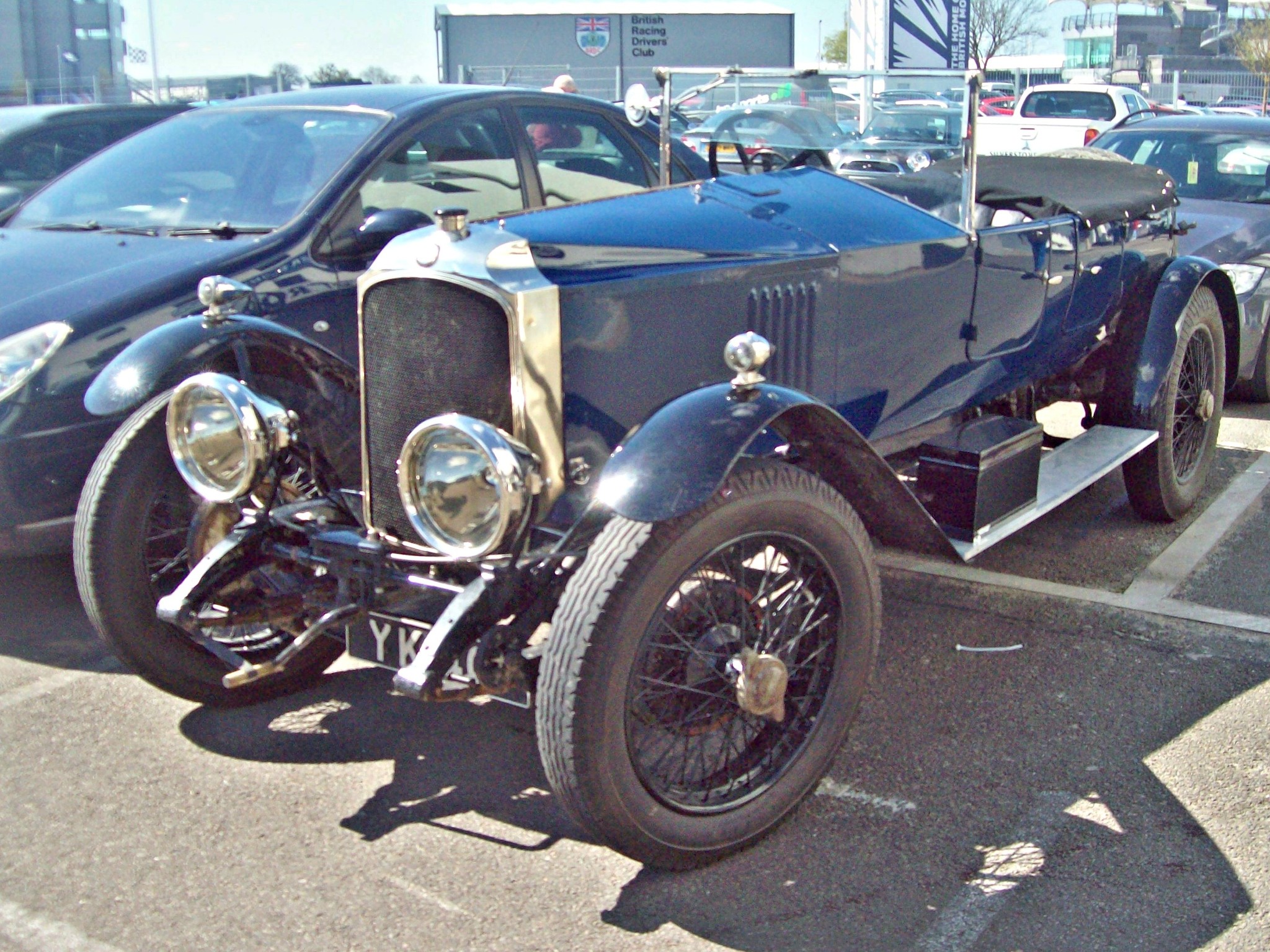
[931, 199, 1034, 230]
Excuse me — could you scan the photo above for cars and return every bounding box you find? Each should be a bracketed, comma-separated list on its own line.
[595, 63, 1270, 183]
[1085, 106, 1270, 405]
[1, 100, 368, 226]
[1, 80, 710, 556]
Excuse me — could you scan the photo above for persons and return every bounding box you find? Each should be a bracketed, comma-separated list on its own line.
[526, 73, 582, 150]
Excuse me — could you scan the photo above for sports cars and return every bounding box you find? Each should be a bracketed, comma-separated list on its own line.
[71, 71, 1242, 873]
